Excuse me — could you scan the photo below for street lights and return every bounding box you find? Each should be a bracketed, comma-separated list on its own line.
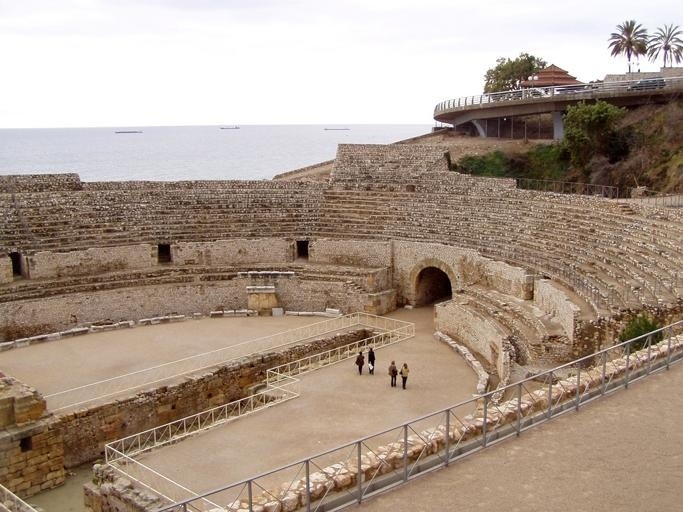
[528, 73, 538, 88]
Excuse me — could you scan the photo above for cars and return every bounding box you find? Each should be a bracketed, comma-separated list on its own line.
[495, 84, 593, 101]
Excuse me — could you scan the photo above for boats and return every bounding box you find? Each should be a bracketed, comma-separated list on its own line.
[324, 128, 351, 130]
[220, 124, 240, 130]
[115, 129, 144, 133]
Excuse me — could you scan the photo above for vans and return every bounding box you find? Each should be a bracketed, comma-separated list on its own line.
[629, 76, 666, 90]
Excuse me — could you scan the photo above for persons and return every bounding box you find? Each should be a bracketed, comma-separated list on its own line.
[388, 360, 397, 388]
[399, 362, 409, 389]
[355, 350, 365, 376]
[366, 347, 375, 375]
